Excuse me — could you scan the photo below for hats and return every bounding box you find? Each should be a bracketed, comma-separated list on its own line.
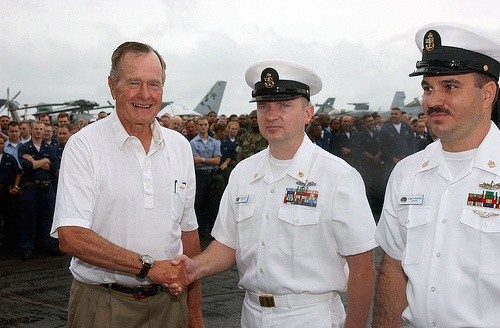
[244, 57, 324, 103]
[409, 22, 500, 82]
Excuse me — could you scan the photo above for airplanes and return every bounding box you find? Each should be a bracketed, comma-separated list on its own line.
[311, 90, 425, 121]
[0, 80, 227, 122]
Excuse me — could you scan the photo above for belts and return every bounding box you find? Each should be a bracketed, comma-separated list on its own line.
[99, 282, 166, 299]
[196, 169, 218, 174]
[245, 289, 336, 308]
[35, 181, 52, 184]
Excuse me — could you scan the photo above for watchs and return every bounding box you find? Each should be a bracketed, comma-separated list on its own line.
[137, 254, 155, 279]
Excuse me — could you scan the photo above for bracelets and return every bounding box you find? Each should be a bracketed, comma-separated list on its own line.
[202, 158, 204, 164]
[15, 186, 20, 190]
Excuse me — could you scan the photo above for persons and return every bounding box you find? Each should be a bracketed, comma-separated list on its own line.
[49, 42, 205, 328]
[373, 20, 500, 328]
[163, 60, 380, 328]
[0, 86, 500, 255]
